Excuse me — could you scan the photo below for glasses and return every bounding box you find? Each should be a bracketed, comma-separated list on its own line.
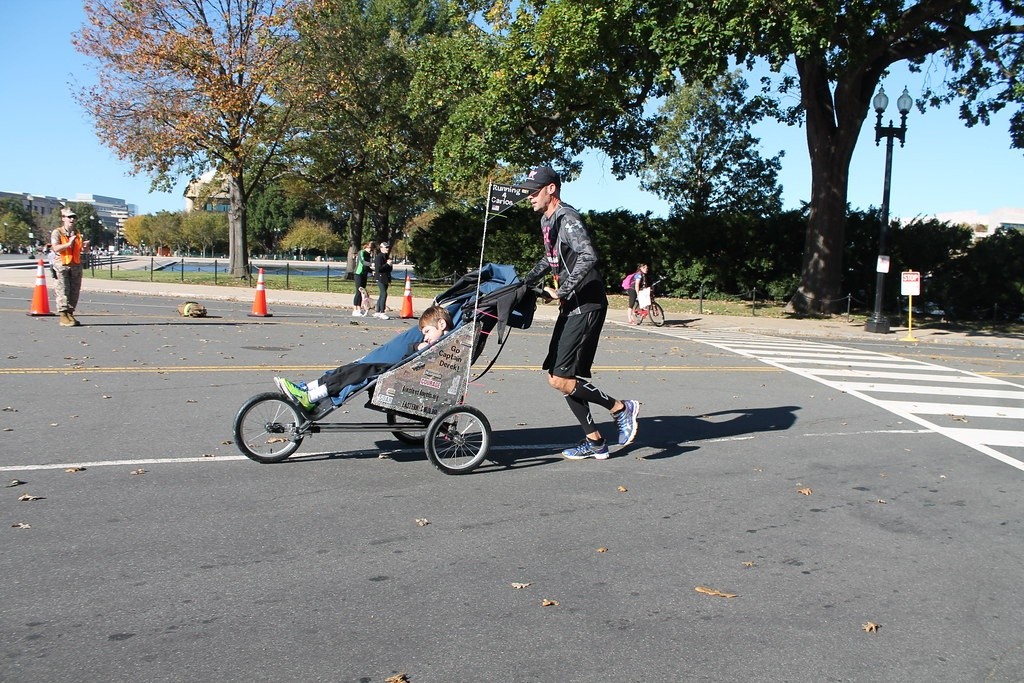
[63, 215, 76, 219]
[382, 246, 389, 249]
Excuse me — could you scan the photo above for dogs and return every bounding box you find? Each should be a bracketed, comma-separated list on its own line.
[175, 302, 207, 316]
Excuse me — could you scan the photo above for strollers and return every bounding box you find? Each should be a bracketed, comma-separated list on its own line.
[230, 266, 552, 471]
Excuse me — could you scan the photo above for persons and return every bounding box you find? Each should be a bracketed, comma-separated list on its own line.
[353, 242, 375, 317]
[627, 264, 648, 325]
[374, 242, 393, 320]
[274, 306, 453, 412]
[51, 210, 91, 327]
[519, 167, 639, 459]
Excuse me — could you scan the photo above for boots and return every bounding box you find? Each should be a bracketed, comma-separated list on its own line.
[66, 310, 80, 325]
[59, 311, 76, 327]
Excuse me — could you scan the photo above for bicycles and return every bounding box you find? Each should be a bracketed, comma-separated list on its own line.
[629, 275, 669, 327]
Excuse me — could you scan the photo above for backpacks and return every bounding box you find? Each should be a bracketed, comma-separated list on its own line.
[622, 272, 637, 290]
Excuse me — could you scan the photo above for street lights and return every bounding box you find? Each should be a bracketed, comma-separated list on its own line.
[98, 220, 103, 248]
[3, 221, 8, 246]
[116, 221, 121, 252]
[185, 241, 189, 256]
[141, 239, 144, 256]
[26, 195, 38, 260]
[89, 214, 95, 251]
[864, 81, 910, 338]
[104, 225, 108, 250]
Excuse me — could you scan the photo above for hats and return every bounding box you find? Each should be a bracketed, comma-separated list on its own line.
[380, 242, 389, 248]
[517, 166, 561, 190]
[61, 208, 77, 217]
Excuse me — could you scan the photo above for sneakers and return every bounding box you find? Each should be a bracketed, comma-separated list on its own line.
[279, 378, 318, 412]
[375, 312, 390, 320]
[274, 376, 308, 398]
[562, 440, 610, 460]
[351, 309, 365, 316]
[610, 399, 639, 446]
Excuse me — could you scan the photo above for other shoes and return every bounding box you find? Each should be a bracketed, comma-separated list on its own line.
[628, 320, 637, 324]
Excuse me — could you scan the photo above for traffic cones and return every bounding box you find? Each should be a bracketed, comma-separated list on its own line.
[246, 268, 274, 318]
[24, 258, 57, 317]
[398, 274, 422, 319]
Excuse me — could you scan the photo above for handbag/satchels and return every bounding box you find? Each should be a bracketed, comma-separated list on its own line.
[636, 287, 651, 309]
